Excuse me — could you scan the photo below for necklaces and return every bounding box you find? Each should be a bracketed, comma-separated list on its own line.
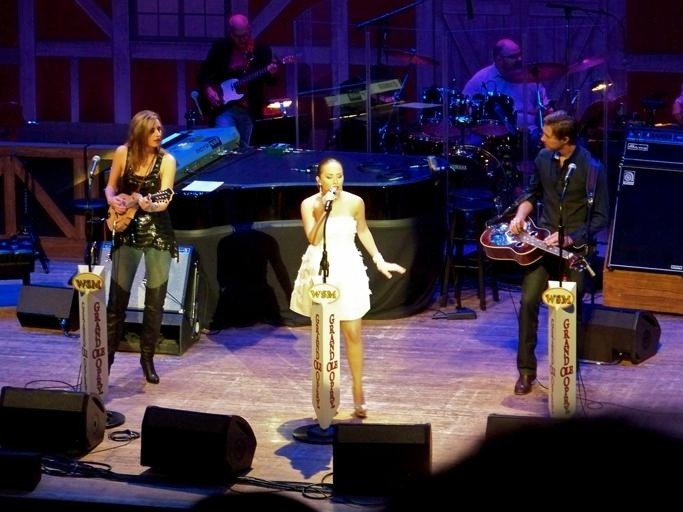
[133, 153, 156, 194]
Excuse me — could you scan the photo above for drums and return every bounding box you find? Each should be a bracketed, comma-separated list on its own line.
[419, 88, 472, 136]
[397, 134, 448, 156]
[469, 92, 518, 135]
[479, 128, 535, 160]
[441, 145, 514, 212]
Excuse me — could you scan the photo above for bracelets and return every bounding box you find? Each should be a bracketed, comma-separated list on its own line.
[148, 202, 161, 212]
[372, 252, 385, 263]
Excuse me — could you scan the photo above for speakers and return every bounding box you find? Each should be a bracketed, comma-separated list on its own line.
[15, 285, 77, 330]
[576, 303, 660, 365]
[1, 386, 106, 456]
[140, 406, 257, 483]
[332, 423, 432, 492]
[485, 413, 550, 437]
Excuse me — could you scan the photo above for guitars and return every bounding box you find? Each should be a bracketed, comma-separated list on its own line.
[480, 213, 594, 274]
[190, 54, 303, 123]
[106, 187, 175, 232]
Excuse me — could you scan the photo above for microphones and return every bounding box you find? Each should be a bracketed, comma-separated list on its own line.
[325, 187, 338, 211]
[88, 155, 102, 177]
[564, 163, 577, 182]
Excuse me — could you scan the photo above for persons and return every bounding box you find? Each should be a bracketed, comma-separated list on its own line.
[671, 92, 683, 126]
[509, 109, 611, 396]
[288, 155, 407, 420]
[460, 36, 551, 129]
[103, 108, 177, 384]
[194, 12, 277, 149]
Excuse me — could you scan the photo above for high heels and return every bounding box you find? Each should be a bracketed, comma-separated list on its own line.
[350, 386, 369, 418]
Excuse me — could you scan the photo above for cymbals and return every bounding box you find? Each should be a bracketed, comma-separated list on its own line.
[388, 49, 440, 65]
[502, 62, 568, 83]
[565, 56, 603, 72]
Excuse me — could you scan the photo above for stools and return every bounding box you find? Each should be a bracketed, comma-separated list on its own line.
[440, 200, 493, 312]
[453, 188, 499, 302]
[0, 236, 35, 285]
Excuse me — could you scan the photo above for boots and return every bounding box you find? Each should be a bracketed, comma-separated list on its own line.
[140, 278, 169, 384]
[106, 277, 130, 378]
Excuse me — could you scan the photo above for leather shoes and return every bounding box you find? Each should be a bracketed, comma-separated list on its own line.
[515, 371, 536, 395]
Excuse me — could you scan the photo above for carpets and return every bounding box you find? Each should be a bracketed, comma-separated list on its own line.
[1, 281, 68, 307]
